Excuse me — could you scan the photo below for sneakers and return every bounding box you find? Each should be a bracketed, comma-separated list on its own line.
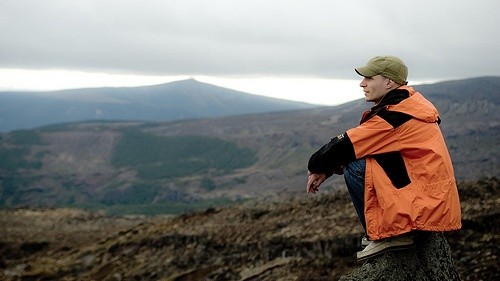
[361, 236, 372, 248]
[356, 235, 416, 261]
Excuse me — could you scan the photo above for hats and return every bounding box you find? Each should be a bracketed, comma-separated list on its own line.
[355, 54, 408, 85]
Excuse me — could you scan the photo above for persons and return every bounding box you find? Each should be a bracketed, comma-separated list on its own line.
[306, 55, 462, 262]
